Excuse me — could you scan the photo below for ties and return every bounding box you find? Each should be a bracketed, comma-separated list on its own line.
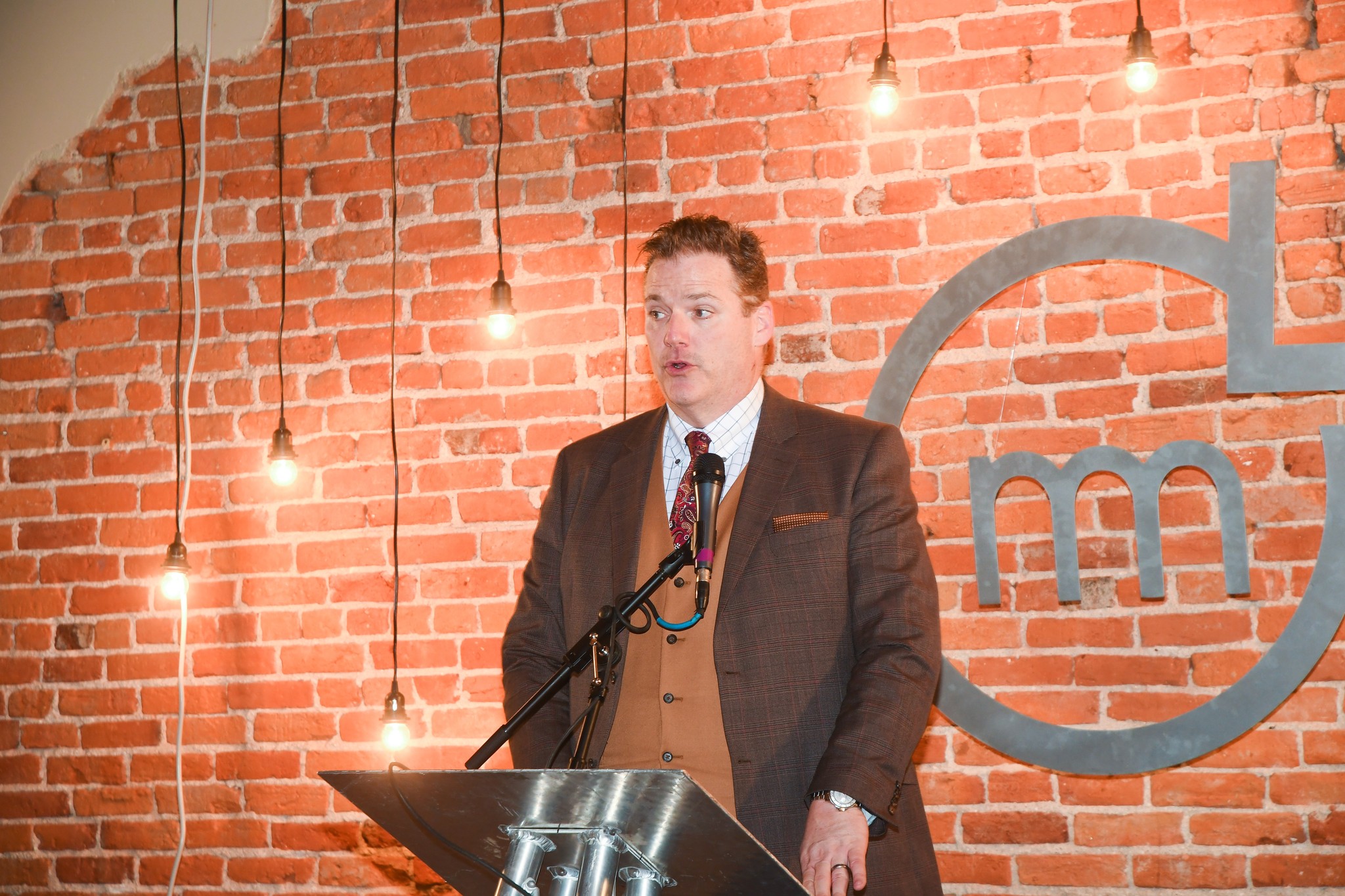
[669, 429, 711, 545]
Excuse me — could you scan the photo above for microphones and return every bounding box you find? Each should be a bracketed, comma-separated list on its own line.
[691, 453, 726, 610]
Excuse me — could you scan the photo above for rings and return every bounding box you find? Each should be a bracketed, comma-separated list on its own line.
[830, 864, 849, 874]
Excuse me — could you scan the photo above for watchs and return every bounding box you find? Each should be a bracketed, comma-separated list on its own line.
[810, 789, 863, 812]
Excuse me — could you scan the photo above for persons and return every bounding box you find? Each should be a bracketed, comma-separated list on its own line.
[498, 214, 946, 896]
